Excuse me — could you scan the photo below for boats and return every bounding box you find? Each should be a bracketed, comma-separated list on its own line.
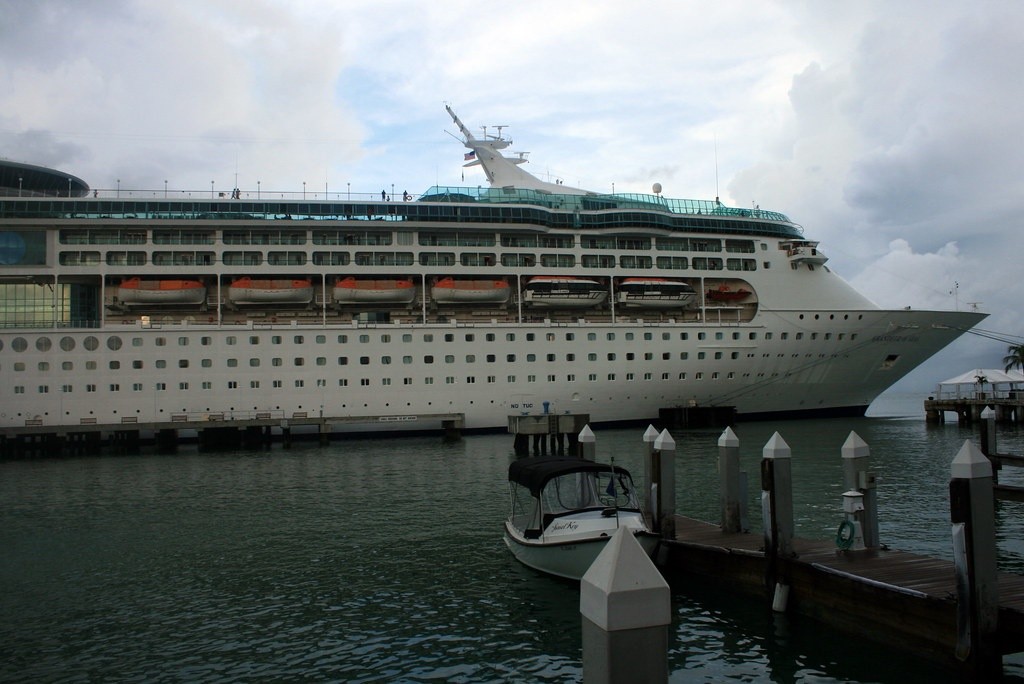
[432, 279, 510, 304]
[0, 104, 993, 431]
[333, 280, 415, 303]
[524, 276, 609, 305]
[228, 280, 312, 302]
[619, 281, 698, 307]
[116, 280, 206, 302]
[501, 454, 660, 578]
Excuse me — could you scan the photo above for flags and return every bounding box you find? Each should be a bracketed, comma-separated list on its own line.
[465, 151, 476, 160]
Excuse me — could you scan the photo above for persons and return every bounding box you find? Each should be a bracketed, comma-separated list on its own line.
[382, 190, 385, 200]
[94, 190, 98, 198]
[404, 191, 407, 201]
[231, 189, 240, 199]
